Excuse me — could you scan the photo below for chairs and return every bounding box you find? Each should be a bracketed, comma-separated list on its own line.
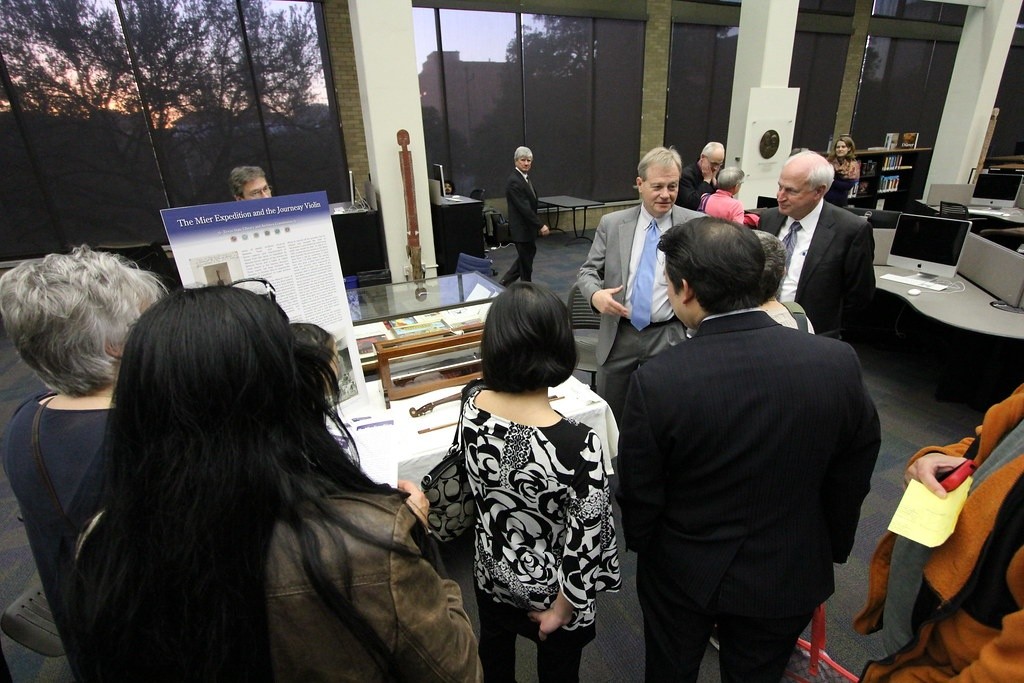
[483, 208, 514, 247]
[940, 200, 1024, 252]
[96, 243, 153, 271]
[567, 281, 602, 393]
[455, 253, 493, 279]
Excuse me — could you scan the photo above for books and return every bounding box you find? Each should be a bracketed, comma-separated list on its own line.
[868, 146, 888, 150]
[848, 155, 902, 198]
[901, 132, 919, 149]
[885, 133, 899, 149]
[390, 312, 483, 335]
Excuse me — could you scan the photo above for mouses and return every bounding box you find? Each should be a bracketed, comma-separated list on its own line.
[1003, 214, 1010, 216]
[907, 289, 921, 295]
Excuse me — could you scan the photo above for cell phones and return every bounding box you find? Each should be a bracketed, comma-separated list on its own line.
[936, 460, 978, 492]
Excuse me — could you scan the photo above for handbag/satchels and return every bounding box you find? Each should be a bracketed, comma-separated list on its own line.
[421, 384, 490, 543]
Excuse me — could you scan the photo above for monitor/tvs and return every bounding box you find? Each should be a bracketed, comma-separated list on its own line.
[887, 214, 973, 283]
[971, 173, 1023, 209]
[433, 163, 445, 197]
[349, 171, 371, 211]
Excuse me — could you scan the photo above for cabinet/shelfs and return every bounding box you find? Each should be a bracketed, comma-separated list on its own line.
[431, 193, 485, 275]
[828, 148, 932, 213]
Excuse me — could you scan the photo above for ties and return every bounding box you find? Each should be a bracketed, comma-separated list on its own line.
[774, 221, 802, 302]
[526, 176, 535, 198]
[630, 219, 658, 331]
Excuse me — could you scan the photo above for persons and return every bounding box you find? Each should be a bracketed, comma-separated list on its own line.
[230, 166, 272, 200]
[675, 142, 725, 211]
[463, 284, 622, 683]
[759, 150, 877, 342]
[854, 383, 1024, 683]
[753, 231, 815, 334]
[498, 147, 550, 287]
[577, 147, 707, 426]
[74, 279, 484, 683]
[701, 167, 745, 224]
[615, 217, 881, 683]
[823, 137, 860, 207]
[0, 252, 173, 683]
[444, 180, 456, 195]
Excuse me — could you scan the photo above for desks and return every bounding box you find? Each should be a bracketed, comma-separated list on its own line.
[322, 356, 621, 576]
[538, 194, 605, 247]
[924, 183, 1024, 237]
[872, 229, 1024, 412]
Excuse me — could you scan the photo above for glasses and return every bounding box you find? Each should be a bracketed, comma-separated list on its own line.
[239, 185, 272, 199]
[227, 278, 276, 304]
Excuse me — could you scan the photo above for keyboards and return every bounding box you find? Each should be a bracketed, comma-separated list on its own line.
[967, 209, 1004, 216]
[881, 274, 948, 291]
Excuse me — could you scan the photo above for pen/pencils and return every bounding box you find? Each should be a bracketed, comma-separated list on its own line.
[417, 421, 458, 434]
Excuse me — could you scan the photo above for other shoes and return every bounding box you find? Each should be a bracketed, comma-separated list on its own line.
[497, 279, 507, 288]
